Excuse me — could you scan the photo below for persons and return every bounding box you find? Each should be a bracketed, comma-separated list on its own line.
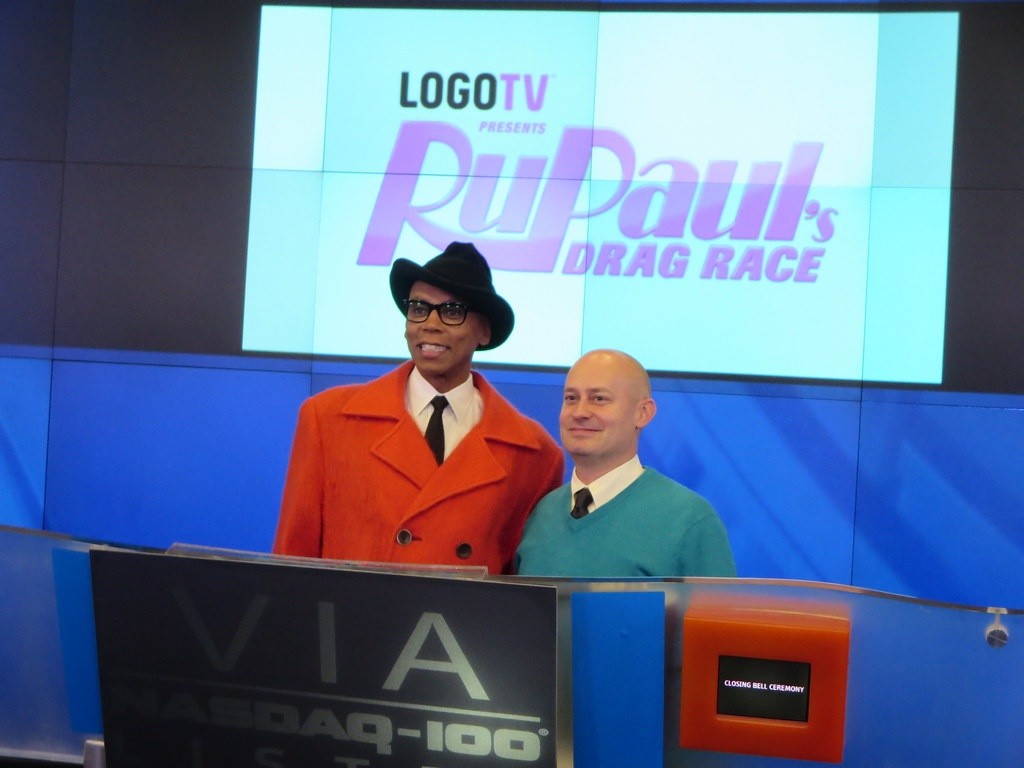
[269, 239, 565, 576]
[507, 348, 739, 579]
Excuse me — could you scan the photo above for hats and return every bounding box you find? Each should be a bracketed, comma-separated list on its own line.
[389, 240, 516, 351]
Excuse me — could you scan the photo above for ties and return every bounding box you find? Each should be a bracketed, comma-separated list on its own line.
[570, 488, 593, 519]
[424, 396, 450, 466]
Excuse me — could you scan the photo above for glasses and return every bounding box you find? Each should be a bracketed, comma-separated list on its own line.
[403, 297, 472, 326]
